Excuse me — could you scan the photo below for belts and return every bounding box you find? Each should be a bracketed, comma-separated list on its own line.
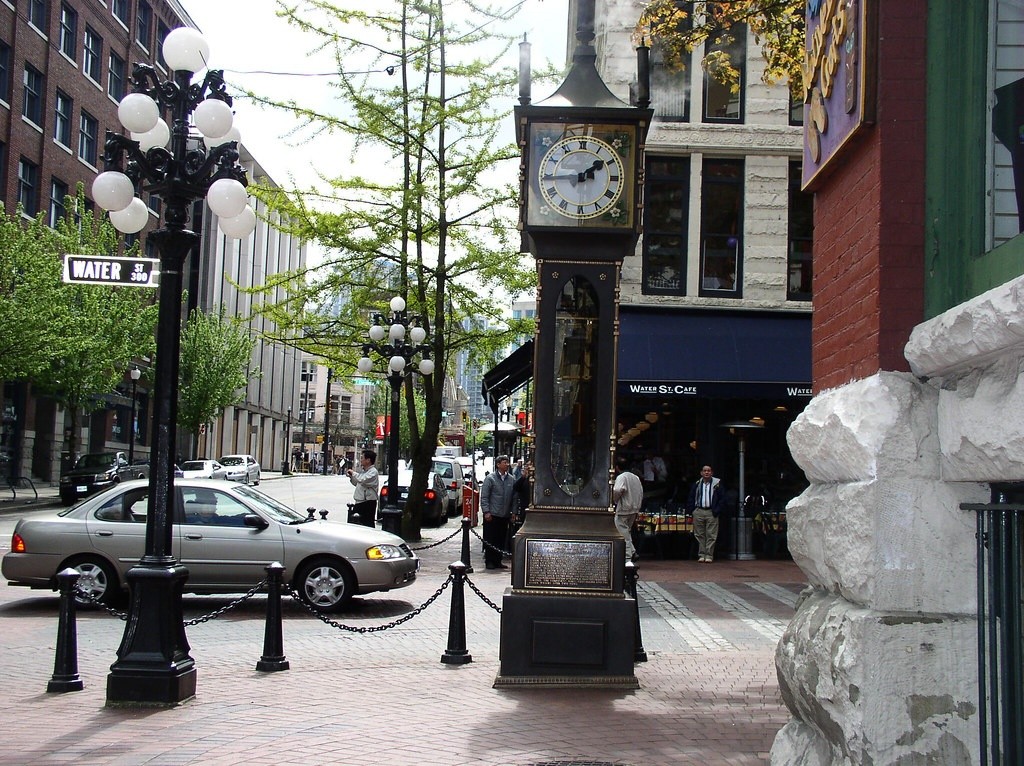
[697, 506, 712, 510]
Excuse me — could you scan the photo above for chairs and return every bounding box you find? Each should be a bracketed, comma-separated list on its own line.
[186, 500, 196, 514]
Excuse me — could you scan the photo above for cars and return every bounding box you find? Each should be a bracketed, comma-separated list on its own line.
[129, 460, 185, 480]
[375, 456, 480, 525]
[0, 478, 422, 614]
[216, 455, 261, 487]
[180, 460, 228, 481]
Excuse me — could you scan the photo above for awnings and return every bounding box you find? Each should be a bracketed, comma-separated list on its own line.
[617, 311, 813, 403]
[482, 338, 534, 414]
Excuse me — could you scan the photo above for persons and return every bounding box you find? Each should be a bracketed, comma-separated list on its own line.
[194, 491, 251, 524]
[295, 448, 302, 468]
[310, 457, 318, 473]
[481, 457, 519, 569]
[690, 464, 724, 562]
[334, 455, 353, 475]
[636, 449, 668, 492]
[612, 458, 643, 562]
[346, 450, 379, 527]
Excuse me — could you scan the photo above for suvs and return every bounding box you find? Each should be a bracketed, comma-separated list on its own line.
[58, 451, 134, 507]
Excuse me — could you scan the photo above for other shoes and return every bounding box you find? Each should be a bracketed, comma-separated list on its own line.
[698, 557, 705, 563]
[486, 564, 509, 569]
[631, 553, 639, 563]
[705, 558, 712, 563]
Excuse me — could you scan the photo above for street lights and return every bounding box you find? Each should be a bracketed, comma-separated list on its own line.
[129, 365, 141, 466]
[282, 405, 293, 476]
[91, 26, 259, 708]
[358, 294, 438, 537]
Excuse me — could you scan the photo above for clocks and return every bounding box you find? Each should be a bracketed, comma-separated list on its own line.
[522, 116, 639, 236]
